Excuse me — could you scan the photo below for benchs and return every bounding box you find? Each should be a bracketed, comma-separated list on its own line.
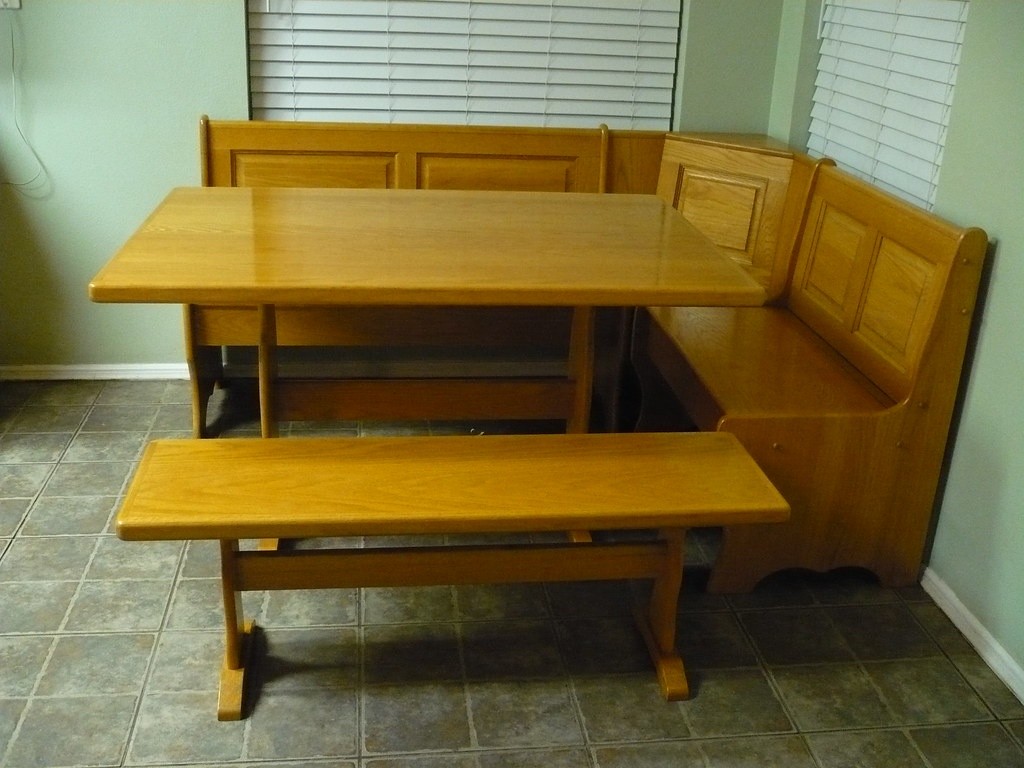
[116, 431, 792, 722]
[181, 114, 628, 439]
[631, 159, 987, 595]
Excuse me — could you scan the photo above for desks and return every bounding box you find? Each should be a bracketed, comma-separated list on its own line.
[87, 186, 765, 550]
